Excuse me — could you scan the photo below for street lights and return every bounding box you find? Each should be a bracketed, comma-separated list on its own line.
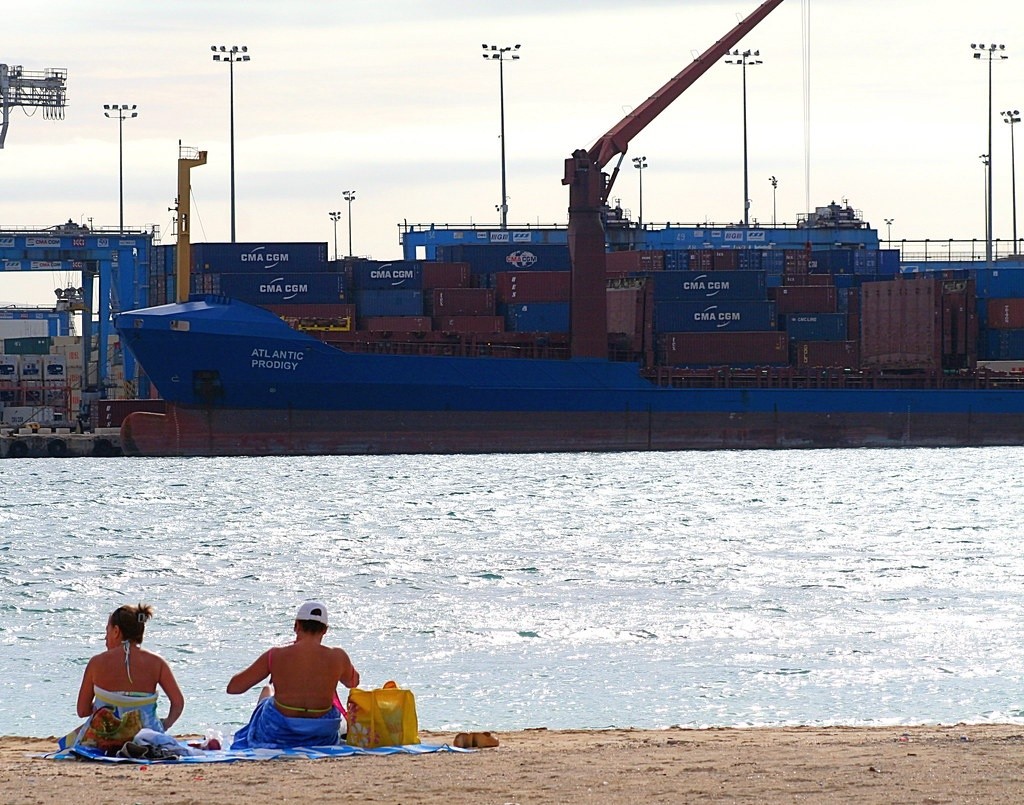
[723, 46, 765, 227]
[884, 218, 894, 251]
[1000, 108, 1022, 255]
[341, 190, 356, 258]
[211, 44, 250, 244]
[769, 177, 777, 229]
[970, 41, 1008, 263]
[481, 42, 522, 229]
[978, 153, 990, 259]
[632, 154, 649, 226]
[103, 103, 138, 234]
[329, 212, 341, 260]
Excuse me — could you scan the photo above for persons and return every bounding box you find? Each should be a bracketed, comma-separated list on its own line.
[226, 601, 361, 750]
[58, 605, 184, 750]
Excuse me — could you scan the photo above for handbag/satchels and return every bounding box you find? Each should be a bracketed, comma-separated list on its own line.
[345, 681, 422, 747]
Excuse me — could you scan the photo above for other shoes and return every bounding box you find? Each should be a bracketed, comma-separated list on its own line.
[452, 732, 474, 748]
[475, 732, 500, 747]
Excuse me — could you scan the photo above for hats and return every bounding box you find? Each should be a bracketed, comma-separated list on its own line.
[295, 601, 329, 626]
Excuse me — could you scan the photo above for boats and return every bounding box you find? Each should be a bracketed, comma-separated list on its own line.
[104, 0, 1024, 449]
